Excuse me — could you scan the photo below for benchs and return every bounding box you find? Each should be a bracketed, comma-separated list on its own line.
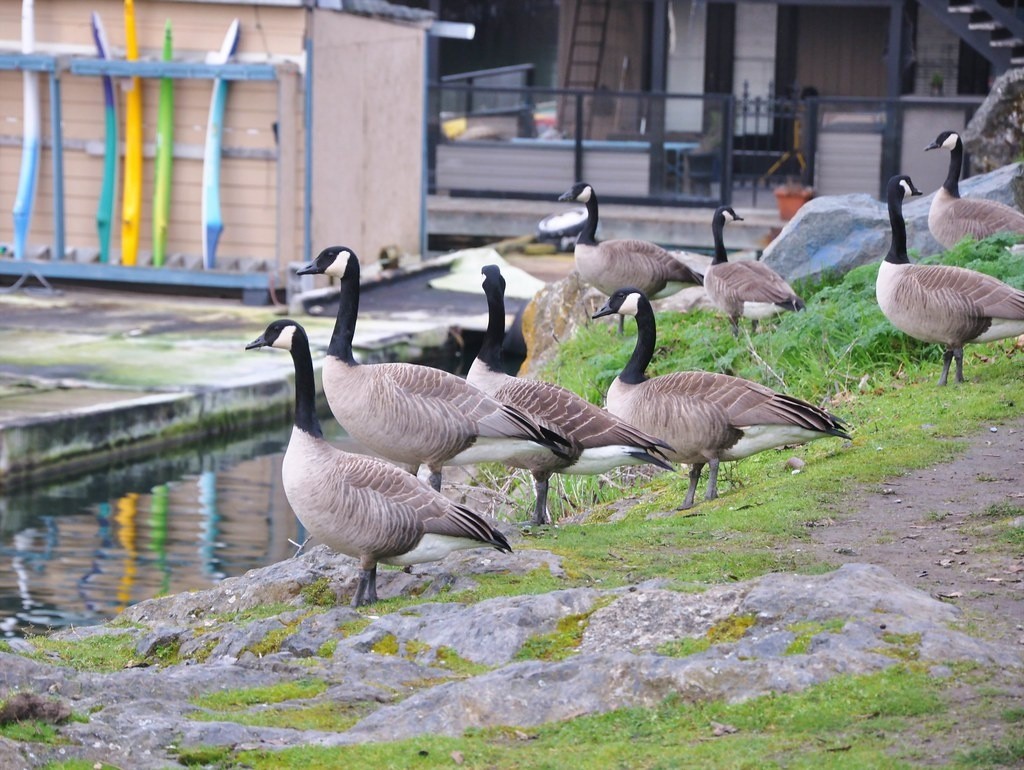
[435, 136, 699, 200]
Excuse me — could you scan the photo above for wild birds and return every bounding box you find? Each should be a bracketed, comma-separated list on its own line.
[295, 247, 573, 494]
[875, 175, 1024, 387]
[704, 206, 807, 350]
[591, 286, 853, 510]
[924, 131, 1023, 251]
[557, 184, 703, 337]
[244, 318, 514, 607]
[465, 265, 677, 524]
